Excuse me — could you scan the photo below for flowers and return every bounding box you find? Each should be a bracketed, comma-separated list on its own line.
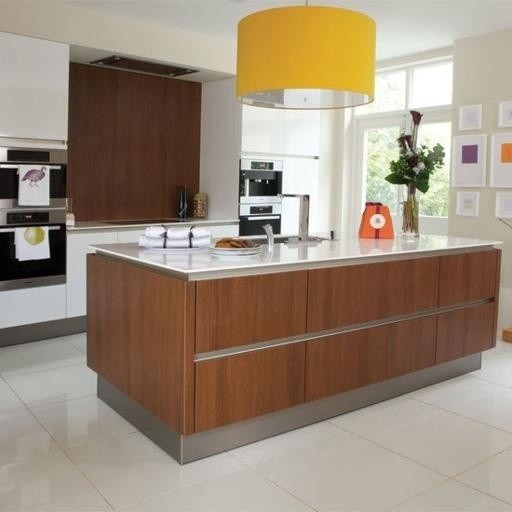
[384, 110, 446, 234]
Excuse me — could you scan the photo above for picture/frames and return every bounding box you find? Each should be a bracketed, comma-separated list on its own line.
[450, 102, 512, 218]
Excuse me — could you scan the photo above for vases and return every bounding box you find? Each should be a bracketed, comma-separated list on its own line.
[396, 181, 424, 245]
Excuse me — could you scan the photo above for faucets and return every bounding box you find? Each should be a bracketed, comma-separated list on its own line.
[274, 191, 310, 240]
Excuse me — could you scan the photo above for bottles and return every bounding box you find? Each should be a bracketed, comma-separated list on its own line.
[192, 193, 208, 218]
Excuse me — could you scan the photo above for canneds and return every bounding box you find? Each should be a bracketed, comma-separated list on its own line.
[193, 194, 209, 219]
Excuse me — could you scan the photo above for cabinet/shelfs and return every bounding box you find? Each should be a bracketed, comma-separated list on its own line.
[0, 30, 69, 150]
[199, 79, 321, 235]
[67, 224, 239, 336]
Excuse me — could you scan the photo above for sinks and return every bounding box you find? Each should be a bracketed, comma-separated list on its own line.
[253, 236, 323, 244]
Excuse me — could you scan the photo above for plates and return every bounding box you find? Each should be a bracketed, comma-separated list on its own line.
[149, 246, 206, 252]
[207, 243, 264, 257]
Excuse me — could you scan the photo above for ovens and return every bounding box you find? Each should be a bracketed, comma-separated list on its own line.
[0, 209, 67, 292]
[0, 136, 69, 208]
[240, 202, 282, 236]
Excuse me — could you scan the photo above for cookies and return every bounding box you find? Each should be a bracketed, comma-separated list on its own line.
[216, 237, 259, 249]
